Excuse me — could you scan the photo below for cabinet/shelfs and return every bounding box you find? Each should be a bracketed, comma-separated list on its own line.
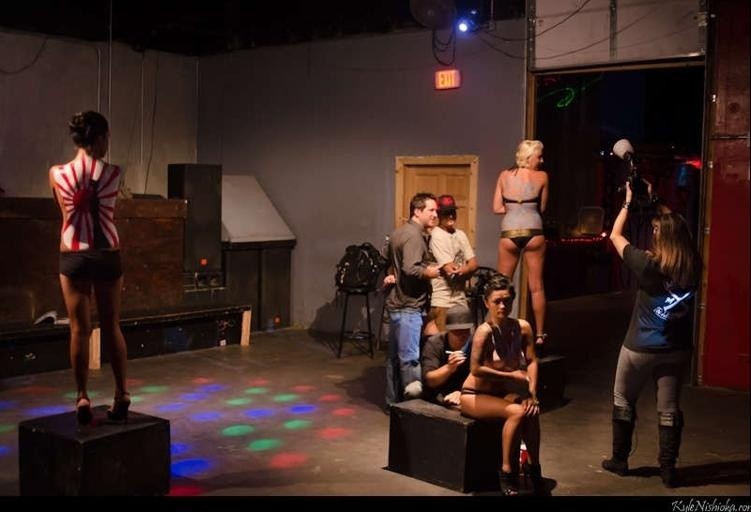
[223, 248, 290, 333]
[0, 195, 188, 330]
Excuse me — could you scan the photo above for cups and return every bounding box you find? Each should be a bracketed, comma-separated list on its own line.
[519, 443, 529, 466]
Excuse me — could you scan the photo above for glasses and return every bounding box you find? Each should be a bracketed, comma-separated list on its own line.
[440, 210, 457, 217]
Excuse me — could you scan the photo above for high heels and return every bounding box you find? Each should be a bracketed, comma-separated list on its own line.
[497, 468, 519, 496]
[521, 460, 545, 492]
[107, 391, 131, 425]
[535, 333, 550, 356]
[75, 396, 94, 426]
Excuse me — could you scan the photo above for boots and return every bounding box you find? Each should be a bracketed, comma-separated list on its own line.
[602, 404, 636, 475]
[658, 410, 679, 487]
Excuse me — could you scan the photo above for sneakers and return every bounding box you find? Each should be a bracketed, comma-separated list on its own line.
[404, 380, 424, 399]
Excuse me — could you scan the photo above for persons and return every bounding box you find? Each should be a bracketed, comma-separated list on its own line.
[602, 179, 704, 488]
[401, 304, 473, 407]
[491, 140, 550, 345]
[460, 273, 554, 497]
[384, 190, 439, 414]
[428, 195, 480, 332]
[45, 109, 133, 422]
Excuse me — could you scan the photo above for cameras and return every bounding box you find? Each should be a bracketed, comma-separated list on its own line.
[616, 154, 647, 196]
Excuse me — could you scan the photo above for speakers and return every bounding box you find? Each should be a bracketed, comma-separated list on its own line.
[167, 163, 222, 278]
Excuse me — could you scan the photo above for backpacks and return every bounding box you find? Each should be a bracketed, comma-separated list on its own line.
[336, 241, 386, 288]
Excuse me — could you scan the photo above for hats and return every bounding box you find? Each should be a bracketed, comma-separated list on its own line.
[438, 195, 459, 209]
[445, 306, 475, 332]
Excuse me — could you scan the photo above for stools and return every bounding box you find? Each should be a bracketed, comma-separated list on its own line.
[388, 399, 519, 494]
[337, 288, 375, 359]
[537, 352, 566, 411]
[17, 405, 171, 498]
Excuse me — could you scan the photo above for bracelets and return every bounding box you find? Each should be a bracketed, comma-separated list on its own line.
[621, 201, 633, 210]
[649, 193, 665, 208]
